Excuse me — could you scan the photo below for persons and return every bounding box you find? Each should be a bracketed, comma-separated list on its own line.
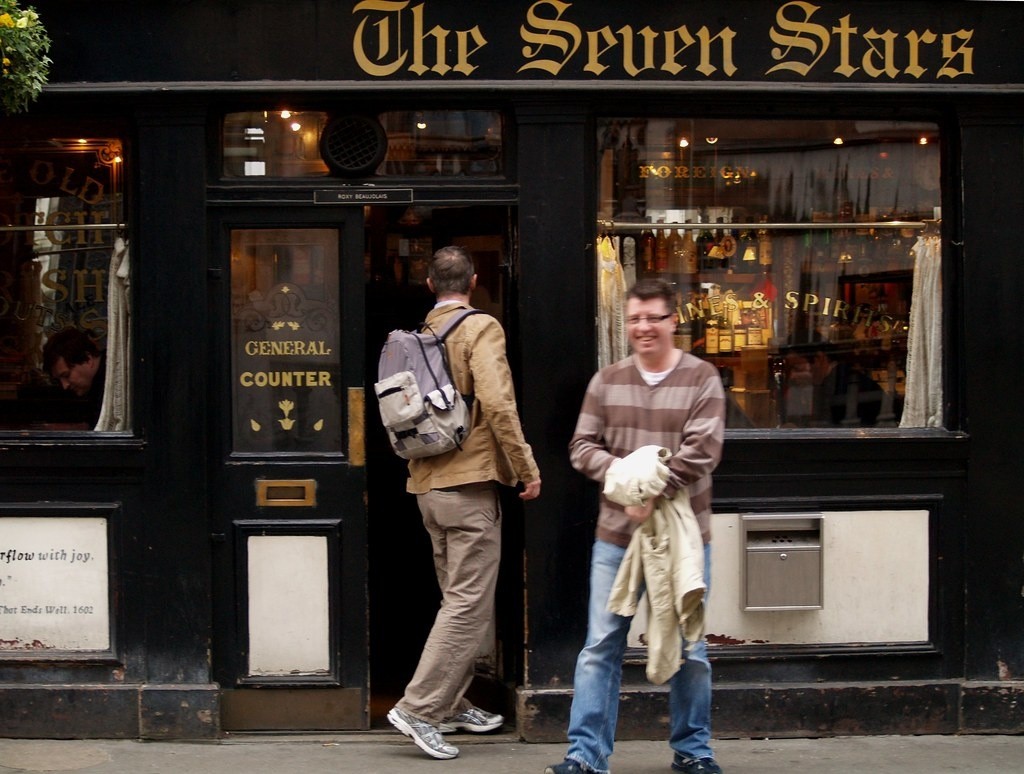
[385, 246, 542, 759]
[544, 279, 725, 774]
[777, 348, 881, 424]
[42, 329, 105, 429]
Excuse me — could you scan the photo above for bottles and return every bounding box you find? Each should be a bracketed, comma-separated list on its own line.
[683, 219, 697, 273]
[654, 220, 667, 272]
[729, 215, 774, 273]
[697, 216, 715, 269]
[667, 222, 683, 272]
[641, 216, 654, 271]
[715, 217, 729, 273]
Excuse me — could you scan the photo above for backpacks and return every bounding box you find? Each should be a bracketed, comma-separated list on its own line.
[372, 308, 485, 460]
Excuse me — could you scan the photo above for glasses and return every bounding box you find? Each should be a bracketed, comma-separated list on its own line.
[624, 311, 673, 324]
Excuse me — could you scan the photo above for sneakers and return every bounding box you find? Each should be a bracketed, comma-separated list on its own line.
[439, 704, 506, 733]
[670, 752, 723, 774]
[386, 706, 460, 760]
[544, 757, 602, 774]
